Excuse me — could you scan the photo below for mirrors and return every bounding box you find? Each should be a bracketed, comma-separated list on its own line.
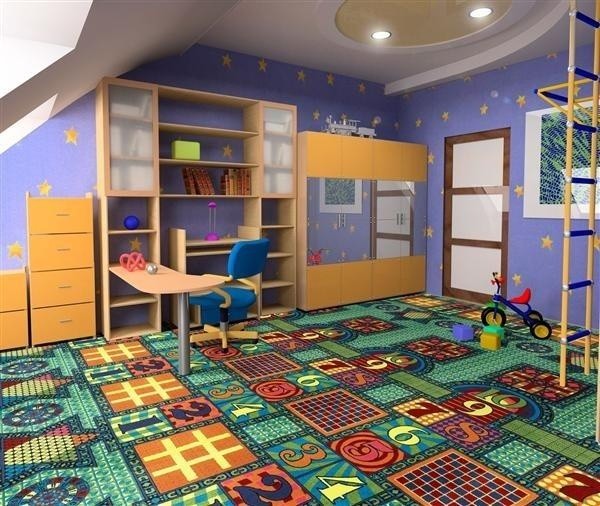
[308, 178, 428, 268]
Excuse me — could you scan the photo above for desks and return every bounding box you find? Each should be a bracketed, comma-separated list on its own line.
[108, 261, 225, 376]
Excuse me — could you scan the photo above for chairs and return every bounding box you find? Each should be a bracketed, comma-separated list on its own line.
[190, 237, 269, 353]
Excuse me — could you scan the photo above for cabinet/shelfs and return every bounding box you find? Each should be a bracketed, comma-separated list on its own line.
[102, 80, 159, 341]
[305, 131, 373, 311]
[243, 99, 299, 318]
[0, 266, 28, 350]
[373, 140, 427, 299]
[26, 192, 96, 347]
[157, 84, 264, 197]
[169, 225, 261, 329]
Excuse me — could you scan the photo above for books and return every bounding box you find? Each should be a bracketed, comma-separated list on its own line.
[182, 166, 251, 195]
